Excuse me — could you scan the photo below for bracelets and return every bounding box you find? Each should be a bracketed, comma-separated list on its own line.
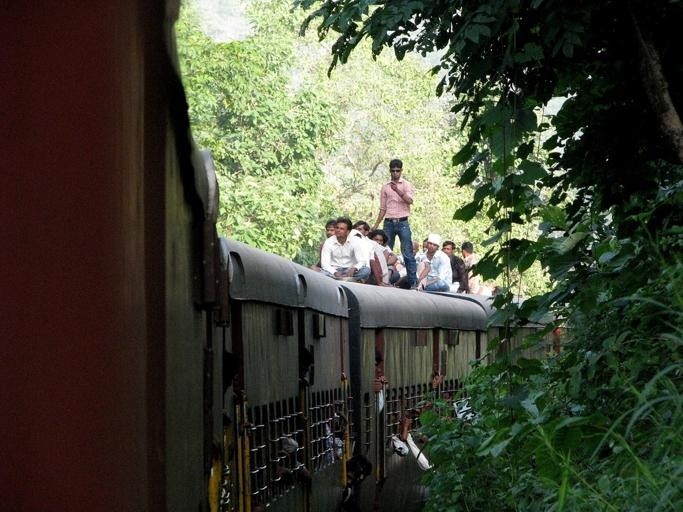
[400, 193, 404, 198]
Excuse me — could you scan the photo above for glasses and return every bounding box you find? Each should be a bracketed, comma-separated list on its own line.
[391, 169, 401, 173]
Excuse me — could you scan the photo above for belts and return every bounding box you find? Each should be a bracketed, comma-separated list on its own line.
[385, 217, 408, 223]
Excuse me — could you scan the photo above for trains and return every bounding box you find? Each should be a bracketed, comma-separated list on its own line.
[4, 2, 570, 508]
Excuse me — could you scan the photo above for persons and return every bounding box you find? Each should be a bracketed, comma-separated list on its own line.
[370, 159, 418, 289]
[221, 344, 459, 512]
[308, 216, 502, 297]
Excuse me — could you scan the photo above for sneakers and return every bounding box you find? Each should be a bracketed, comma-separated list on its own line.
[411, 283, 418, 290]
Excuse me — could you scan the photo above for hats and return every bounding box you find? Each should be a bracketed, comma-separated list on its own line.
[368, 229, 388, 243]
[428, 234, 442, 246]
